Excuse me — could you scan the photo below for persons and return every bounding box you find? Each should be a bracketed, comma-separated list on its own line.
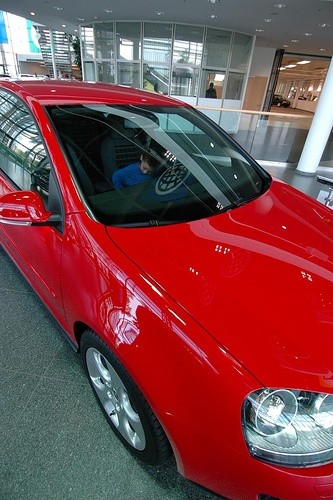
[112, 149, 158, 190]
[205, 82, 217, 99]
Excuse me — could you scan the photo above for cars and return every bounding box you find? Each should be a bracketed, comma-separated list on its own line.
[1, 78, 332, 499]
[270, 93, 291, 107]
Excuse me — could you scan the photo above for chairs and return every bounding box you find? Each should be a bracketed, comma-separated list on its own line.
[102, 127, 142, 184]
[66, 118, 104, 176]
